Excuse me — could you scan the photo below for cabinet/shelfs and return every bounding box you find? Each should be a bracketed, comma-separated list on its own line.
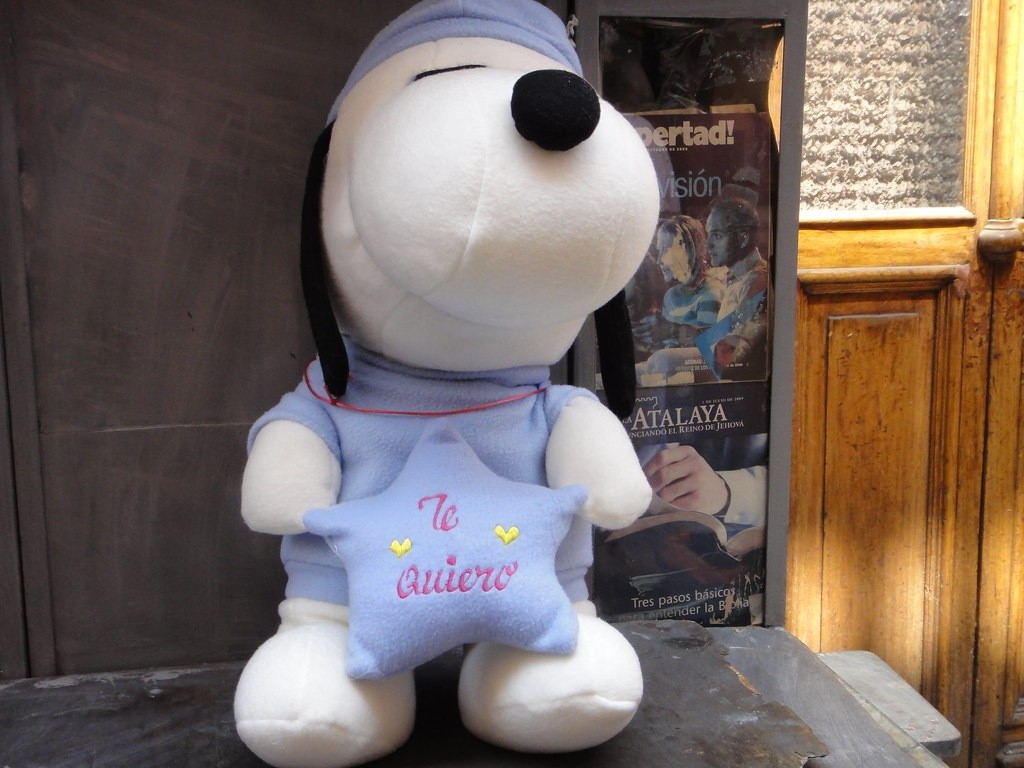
[785, 0, 1024, 768]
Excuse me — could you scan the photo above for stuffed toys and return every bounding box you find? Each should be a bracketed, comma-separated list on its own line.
[233, 0, 661, 768]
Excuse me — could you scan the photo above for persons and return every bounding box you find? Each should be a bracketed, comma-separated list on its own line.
[595, 433, 767, 626]
[625, 197, 768, 384]
